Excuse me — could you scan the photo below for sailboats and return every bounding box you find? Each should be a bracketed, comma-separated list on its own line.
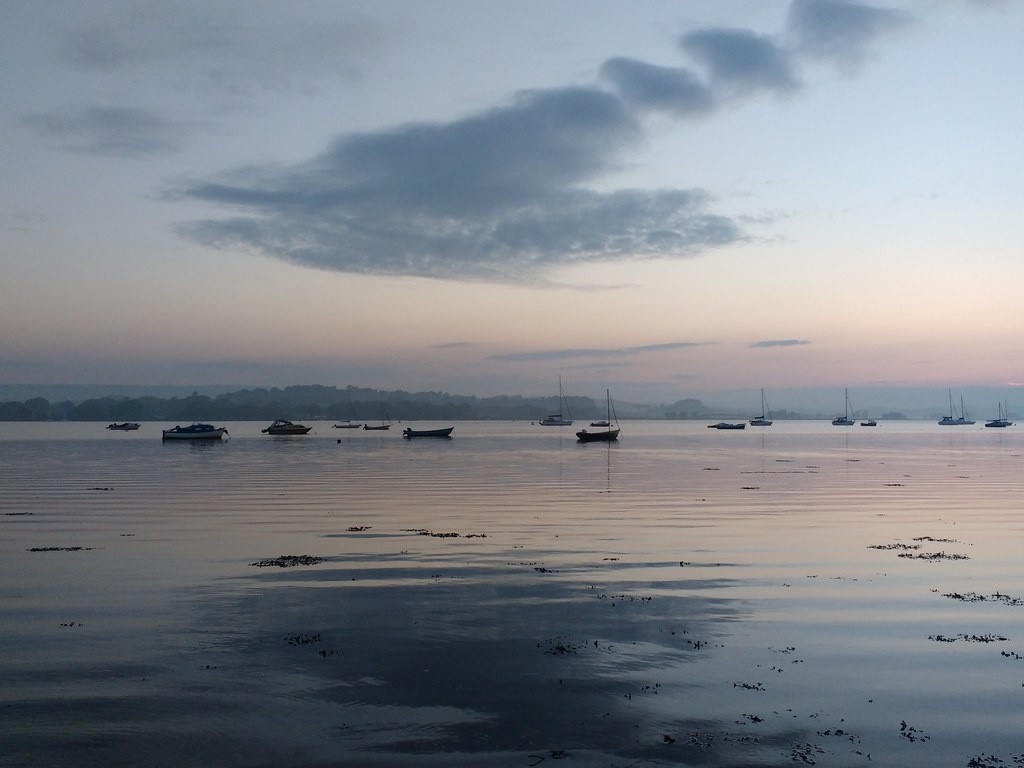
[937, 388, 960, 425]
[537, 376, 573, 426]
[575, 388, 621, 443]
[832, 387, 856, 425]
[748, 388, 773, 427]
[984, 399, 1013, 428]
[958, 395, 976, 425]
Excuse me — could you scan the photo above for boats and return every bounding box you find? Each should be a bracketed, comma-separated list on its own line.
[861, 419, 878, 426]
[262, 418, 313, 435]
[363, 423, 390, 431]
[403, 427, 455, 440]
[589, 422, 610, 427]
[716, 423, 747, 429]
[106, 423, 141, 432]
[162, 422, 231, 443]
[332, 424, 362, 428]
